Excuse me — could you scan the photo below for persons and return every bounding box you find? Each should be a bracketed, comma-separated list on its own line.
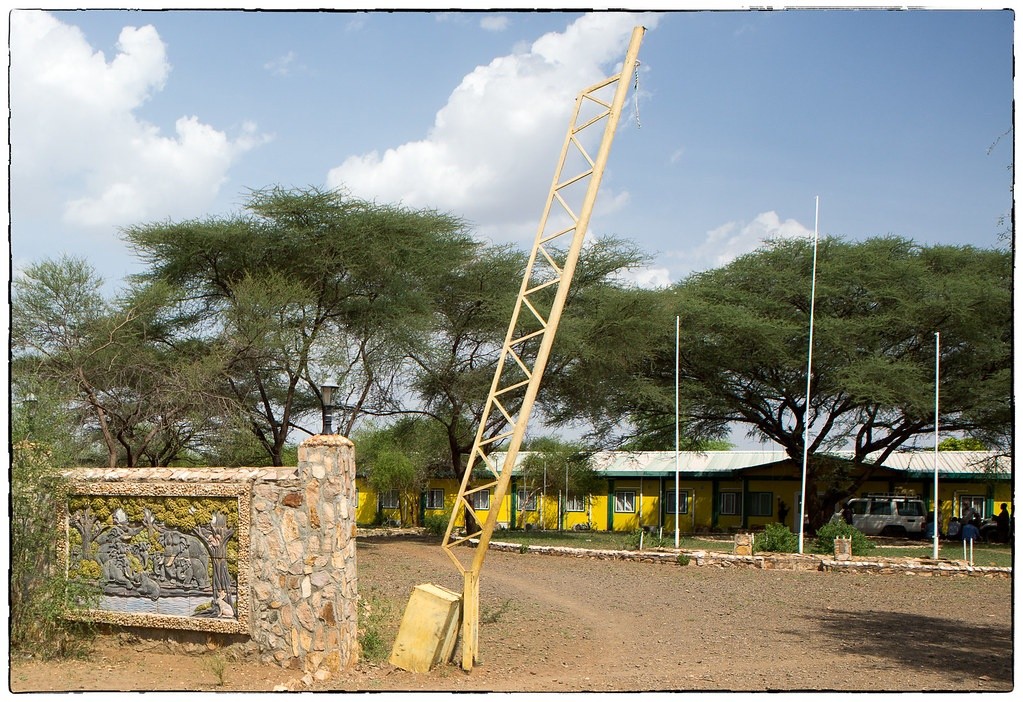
[925, 498, 982, 544]
[992, 503, 1009, 543]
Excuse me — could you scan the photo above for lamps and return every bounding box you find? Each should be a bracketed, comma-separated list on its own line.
[320, 377, 340, 406]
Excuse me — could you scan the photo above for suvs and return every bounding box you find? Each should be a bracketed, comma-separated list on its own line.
[804, 494, 928, 538]
